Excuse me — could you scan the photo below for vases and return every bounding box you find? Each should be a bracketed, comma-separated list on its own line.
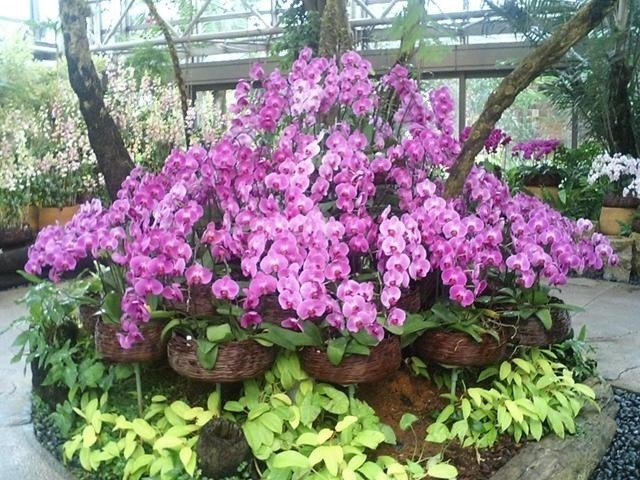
[602, 188, 638, 207]
[196, 417, 249, 479]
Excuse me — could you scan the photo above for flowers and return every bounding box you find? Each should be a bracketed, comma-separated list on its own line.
[584, 153, 640, 199]
[1, 42, 620, 372]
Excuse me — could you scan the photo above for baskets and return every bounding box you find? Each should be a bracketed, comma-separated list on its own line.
[300, 333, 404, 386]
[165, 285, 229, 322]
[475, 295, 573, 350]
[371, 271, 437, 322]
[256, 287, 335, 333]
[94, 314, 166, 363]
[524, 174, 561, 186]
[167, 328, 280, 383]
[602, 190, 639, 209]
[411, 319, 507, 371]
[79, 291, 100, 334]
